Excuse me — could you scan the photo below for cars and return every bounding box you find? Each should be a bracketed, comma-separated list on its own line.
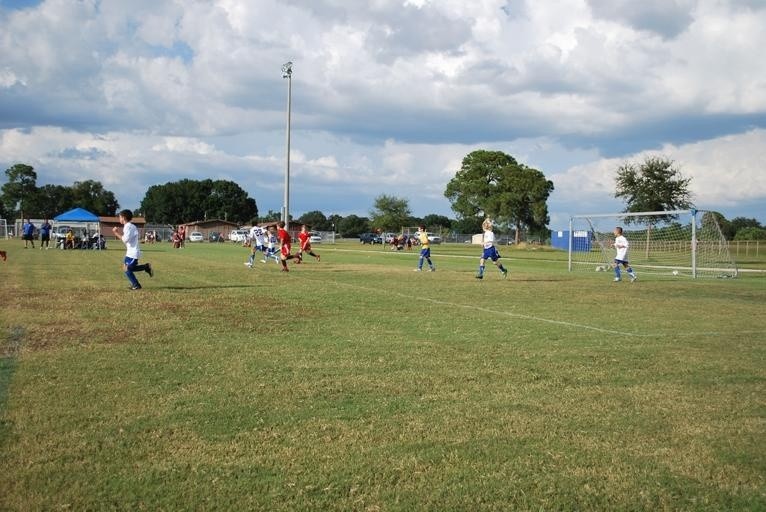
[359, 231, 442, 246]
[307, 232, 321, 244]
[188, 229, 250, 243]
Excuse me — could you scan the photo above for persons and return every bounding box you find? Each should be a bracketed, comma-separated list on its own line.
[611, 227, 638, 283]
[39, 217, 52, 250]
[112, 209, 153, 290]
[96, 234, 106, 249]
[412, 224, 436, 272]
[64, 229, 76, 249]
[473, 219, 507, 278]
[242, 220, 320, 272]
[177, 226, 185, 248]
[172, 229, 181, 248]
[21, 216, 35, 248]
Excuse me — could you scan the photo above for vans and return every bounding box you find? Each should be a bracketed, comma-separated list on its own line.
[55, 225, 73, 242]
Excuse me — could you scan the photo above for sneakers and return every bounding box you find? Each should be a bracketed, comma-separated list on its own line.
[260, 257, 279, 264]
[475, 275, 482, 278]
[612, 277, 622, 282]
[145, 263, 153, 277]
[244, 262, 252, 268]
[414, 268, 435, 273]
[502, 269, 507, 279]
[128, 284, 142, 291]
[282, 253, 302, 272]
[630, 276, 637, 283]
[316, 255, 320, 262]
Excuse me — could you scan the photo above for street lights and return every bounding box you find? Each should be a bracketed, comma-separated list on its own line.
[281, 62, 293, 233]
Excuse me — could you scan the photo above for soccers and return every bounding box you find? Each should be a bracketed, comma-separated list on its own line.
[673, 270, 679, 276]
